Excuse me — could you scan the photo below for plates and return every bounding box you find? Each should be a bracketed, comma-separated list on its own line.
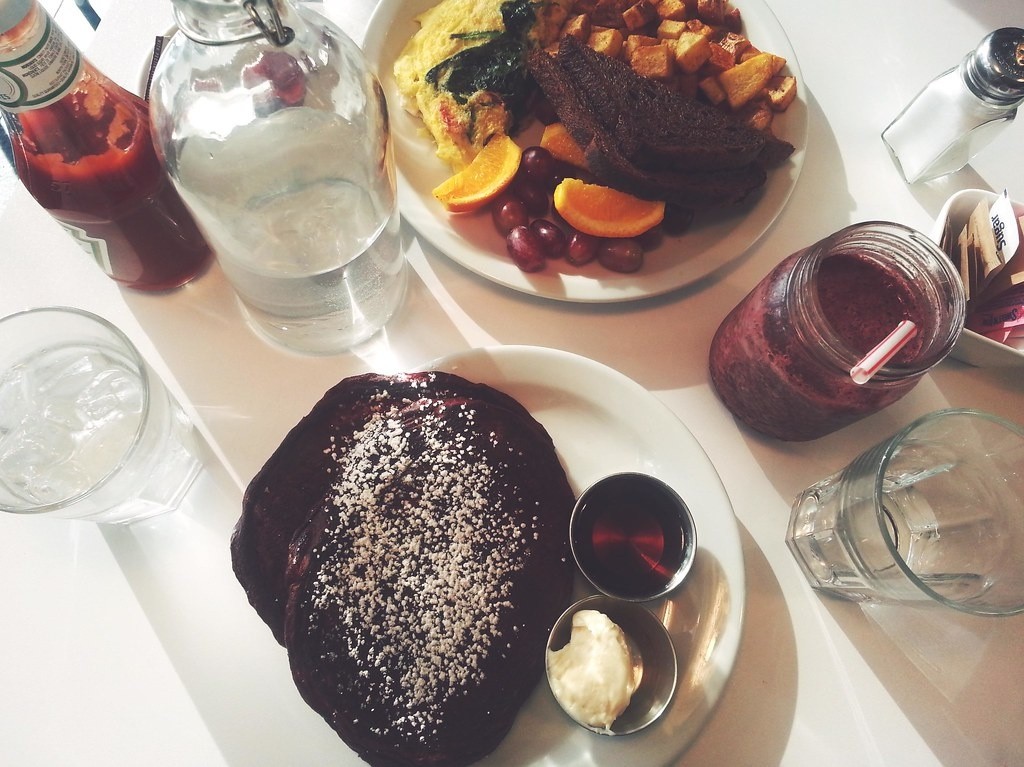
[287, 344, 745, 767]
[360, 0, 809, 304]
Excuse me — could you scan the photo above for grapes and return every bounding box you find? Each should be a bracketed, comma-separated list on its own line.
[491, 146, 651, 272]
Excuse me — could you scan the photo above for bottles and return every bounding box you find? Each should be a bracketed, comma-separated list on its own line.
[147, 0, 407, 355]
[881, 28, 1024, 185]
[2, 0, 213, 296]
[708, 220, 966, 443]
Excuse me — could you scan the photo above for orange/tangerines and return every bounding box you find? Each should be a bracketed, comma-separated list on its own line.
[432, 132, 522, 212]
[555, 177, 665, 238]
[540, 122, 589, 167]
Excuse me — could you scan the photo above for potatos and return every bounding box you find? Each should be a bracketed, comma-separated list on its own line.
[539, 0, 798, 131]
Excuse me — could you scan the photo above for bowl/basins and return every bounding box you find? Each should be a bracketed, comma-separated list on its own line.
[926, 188, 1024, 370]
[569, 470, 699, 603]
[543, 596, 678, 736]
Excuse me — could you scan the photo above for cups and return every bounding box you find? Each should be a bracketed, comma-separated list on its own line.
[1, 305, 204, 526]
[784, 407, 1024, 617]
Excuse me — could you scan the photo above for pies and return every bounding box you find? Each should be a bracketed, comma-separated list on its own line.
[391, 0, 578, 169]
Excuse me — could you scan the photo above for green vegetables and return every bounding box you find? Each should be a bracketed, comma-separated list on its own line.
[422, 0, 560, 144]
